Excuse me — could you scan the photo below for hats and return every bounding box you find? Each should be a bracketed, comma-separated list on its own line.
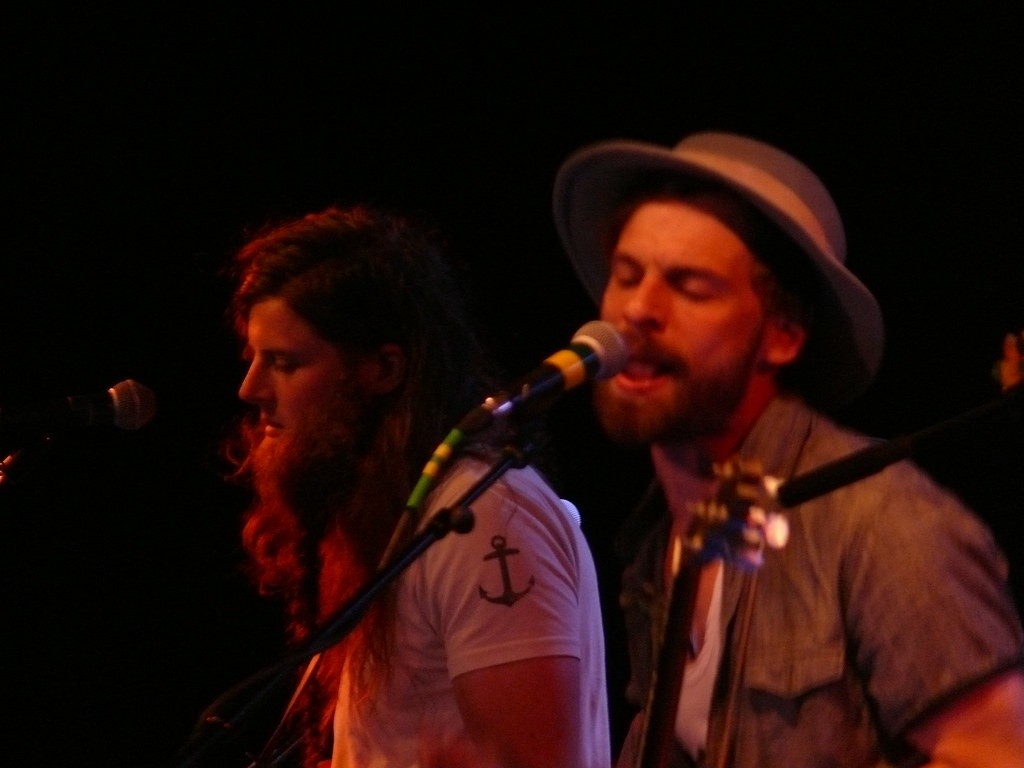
[551, 132, 884, 414]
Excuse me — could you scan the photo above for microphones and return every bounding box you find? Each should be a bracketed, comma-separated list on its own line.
[1, 378, 156, 474]
[455, 321, 627, 438]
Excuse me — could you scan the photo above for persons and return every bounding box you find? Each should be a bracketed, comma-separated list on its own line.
[222, 208, 610, 768]
[554, 132, 1023, 768]
